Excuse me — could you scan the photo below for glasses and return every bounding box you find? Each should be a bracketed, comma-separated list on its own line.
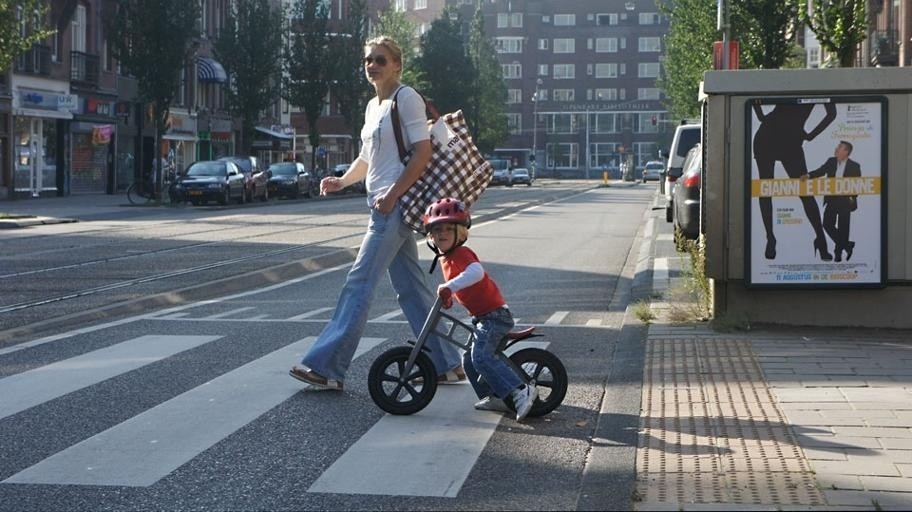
[362, 57, 386, 65]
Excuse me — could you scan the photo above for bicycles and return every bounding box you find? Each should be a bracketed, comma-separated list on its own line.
[367, 288, 568, 417]
[127, 165, 189, 207]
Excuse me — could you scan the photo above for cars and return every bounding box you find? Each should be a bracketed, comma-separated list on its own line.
[511, 168, 531, 187]
[168, 154, 367, 206]
[667, 142, 701, 243]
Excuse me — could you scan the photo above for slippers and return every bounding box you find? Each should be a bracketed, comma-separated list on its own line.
[412, 371, 466, 385]
[289, 369, 343, 390]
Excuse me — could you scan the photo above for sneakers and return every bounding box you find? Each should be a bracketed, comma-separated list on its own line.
[511, 384, 539, 422]
[474, 395, 513, 413]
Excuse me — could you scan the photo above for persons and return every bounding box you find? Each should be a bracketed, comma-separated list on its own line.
[800, 138, 863, 263]
[423, 197, 541, 425]
[285, 33, 469, 394]
[751, 98, 838, 264]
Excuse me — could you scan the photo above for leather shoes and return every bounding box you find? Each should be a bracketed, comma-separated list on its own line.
[834, 246, 842, 261]
[845, 241, 855, 260]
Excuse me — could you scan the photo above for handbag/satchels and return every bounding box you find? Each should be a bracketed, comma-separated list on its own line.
[398, 111, 494, 236]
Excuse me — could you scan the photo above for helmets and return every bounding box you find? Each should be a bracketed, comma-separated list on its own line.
[424, 197, 471, 231]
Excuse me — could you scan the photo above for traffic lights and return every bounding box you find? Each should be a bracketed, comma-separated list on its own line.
[652, 115, 656, 125]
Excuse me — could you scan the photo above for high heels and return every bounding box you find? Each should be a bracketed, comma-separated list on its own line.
[814, 238, 833, 260]
[766, 239, 776, 259]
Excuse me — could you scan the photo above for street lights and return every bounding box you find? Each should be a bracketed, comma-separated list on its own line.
[531, 79, 545, 181]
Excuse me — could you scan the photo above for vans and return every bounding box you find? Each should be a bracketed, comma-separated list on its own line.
[642, 161, 665, 183]
[485, 159, 512, 187]
[659, 119, 701, 222]
